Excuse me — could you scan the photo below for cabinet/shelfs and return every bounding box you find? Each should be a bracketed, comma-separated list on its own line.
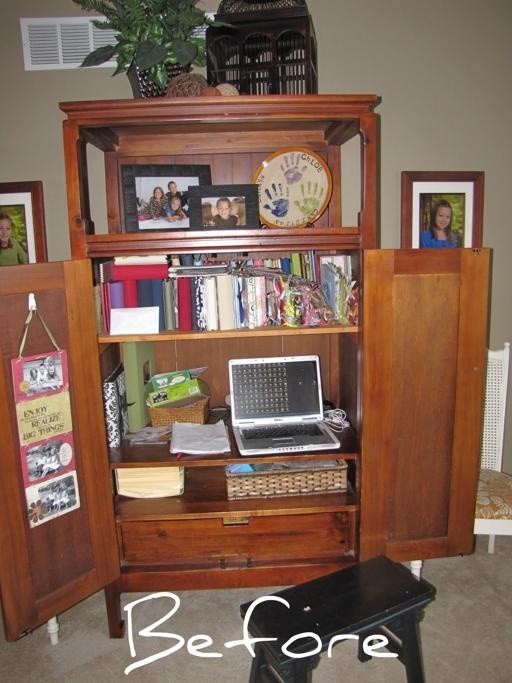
[0, 92, 492, 643]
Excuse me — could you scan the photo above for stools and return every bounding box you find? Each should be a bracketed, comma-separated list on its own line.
[240, 553, 436, 682]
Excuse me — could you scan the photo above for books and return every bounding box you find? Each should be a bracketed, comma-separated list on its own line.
[91, 255, 354, 335]
[168, 418, 231, 455]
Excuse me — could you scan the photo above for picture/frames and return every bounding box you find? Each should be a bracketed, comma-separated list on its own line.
[0, 180, 49, 265]
[119, 163, 212, 235]
[189, 182, 261, 230]
[401, 168, 487, 251]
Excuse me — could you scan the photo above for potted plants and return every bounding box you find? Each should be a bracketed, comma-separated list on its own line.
[74, 0, 238, 99]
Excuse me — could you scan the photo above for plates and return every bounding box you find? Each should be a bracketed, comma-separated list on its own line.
[251, 145, 333, 229]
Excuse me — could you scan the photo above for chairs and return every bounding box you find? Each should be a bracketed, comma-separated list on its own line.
[409, 342, 512, 581]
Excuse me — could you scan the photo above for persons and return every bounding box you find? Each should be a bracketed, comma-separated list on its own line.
[47, 483, 69, 510]
[202, 198, 244, 225]
[139, 182, 188, 222]
[0, 214, 28, 264]
[38, 449, 59, 475]
[28, 365, 59, 389]
[420, 198, 459, 248]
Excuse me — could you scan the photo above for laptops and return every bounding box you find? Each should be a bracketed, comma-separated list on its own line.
[227, 354, 340, 458]
